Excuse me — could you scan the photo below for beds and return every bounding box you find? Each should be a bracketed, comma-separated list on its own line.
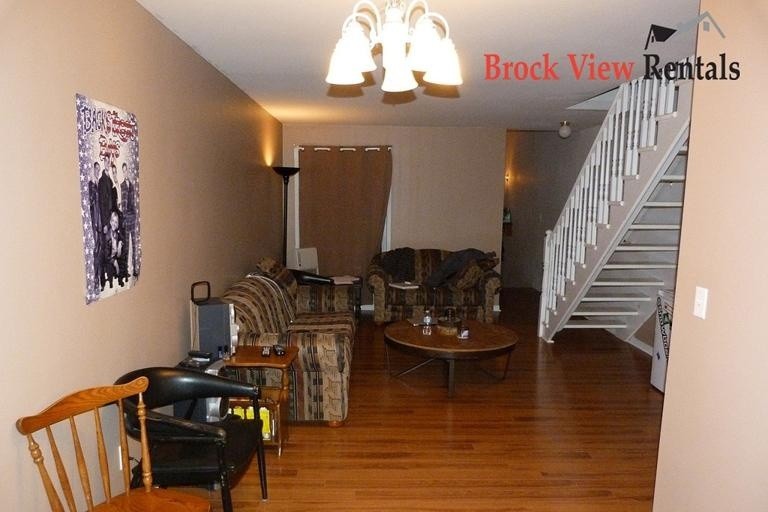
[383, 319, 519, 398]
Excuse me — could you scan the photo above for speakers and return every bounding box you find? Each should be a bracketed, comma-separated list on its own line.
[190, 297, 240, 360]
[173, 357, 229, 423]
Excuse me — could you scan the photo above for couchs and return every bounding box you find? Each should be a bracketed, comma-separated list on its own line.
[365, 247, 504, 326]
[221, 255, 358, 427]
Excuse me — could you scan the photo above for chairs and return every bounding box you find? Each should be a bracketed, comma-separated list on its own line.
[16, 376, 213, 512]
[112, 366, 267, 511]
[295, 246, 319, 276]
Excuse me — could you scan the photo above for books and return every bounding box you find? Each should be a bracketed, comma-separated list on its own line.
[388, 281, 420, 290]
[330, 275, 360, 286]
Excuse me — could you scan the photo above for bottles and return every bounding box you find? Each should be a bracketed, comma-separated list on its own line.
[423, 310, 432, 335]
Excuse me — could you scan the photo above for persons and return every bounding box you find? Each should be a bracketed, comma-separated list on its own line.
[88, 154, 138, 292]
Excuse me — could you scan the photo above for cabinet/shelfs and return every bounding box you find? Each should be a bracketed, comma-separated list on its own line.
[650, 288, 675, 393]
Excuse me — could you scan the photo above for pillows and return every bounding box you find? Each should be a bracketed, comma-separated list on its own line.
[447, 254, 483, 291]
[257, 257, 297, 298]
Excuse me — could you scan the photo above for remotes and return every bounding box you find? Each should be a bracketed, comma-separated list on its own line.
[273, 344, 285, 356]
[188, 351, 212, 358]
[192, 357, 210, 362]
[261, 346, 271, 357]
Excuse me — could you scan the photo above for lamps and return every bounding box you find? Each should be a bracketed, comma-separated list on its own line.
[558, 120, 572, 140]
[325, 1, 464, 93]
[272, 166, 301, 267]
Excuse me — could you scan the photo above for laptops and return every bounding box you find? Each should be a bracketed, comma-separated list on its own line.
[406, 318, 439, 327]
